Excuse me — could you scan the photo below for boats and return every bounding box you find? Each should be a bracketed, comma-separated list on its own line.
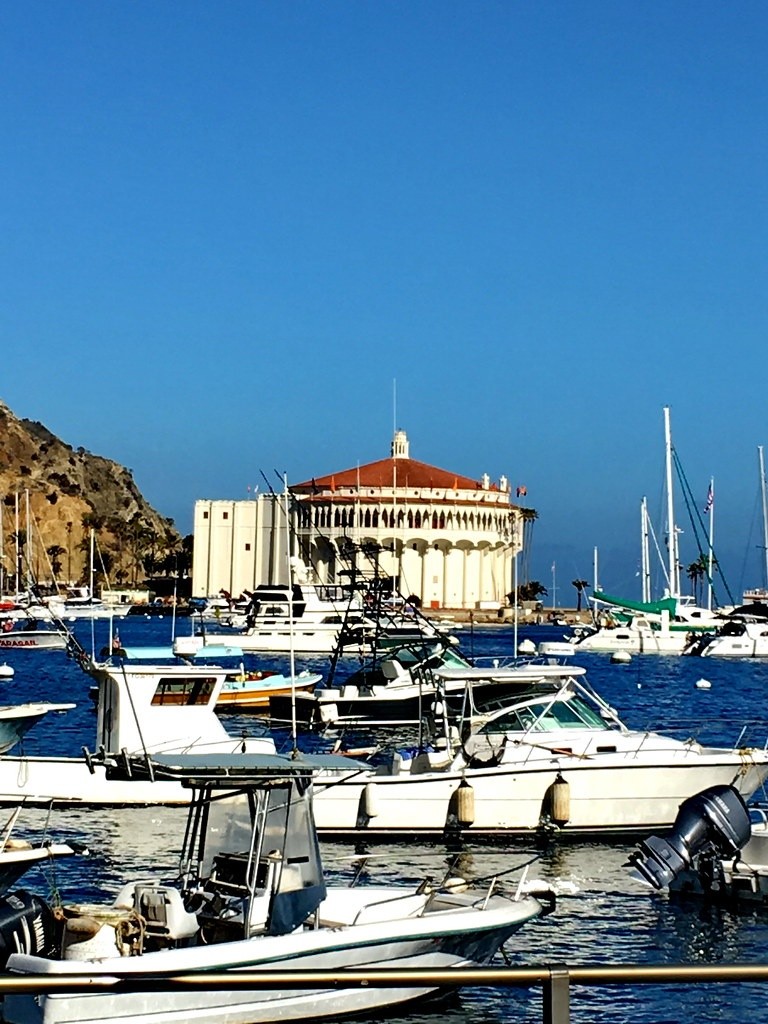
[0, 406, 768, 1024]
